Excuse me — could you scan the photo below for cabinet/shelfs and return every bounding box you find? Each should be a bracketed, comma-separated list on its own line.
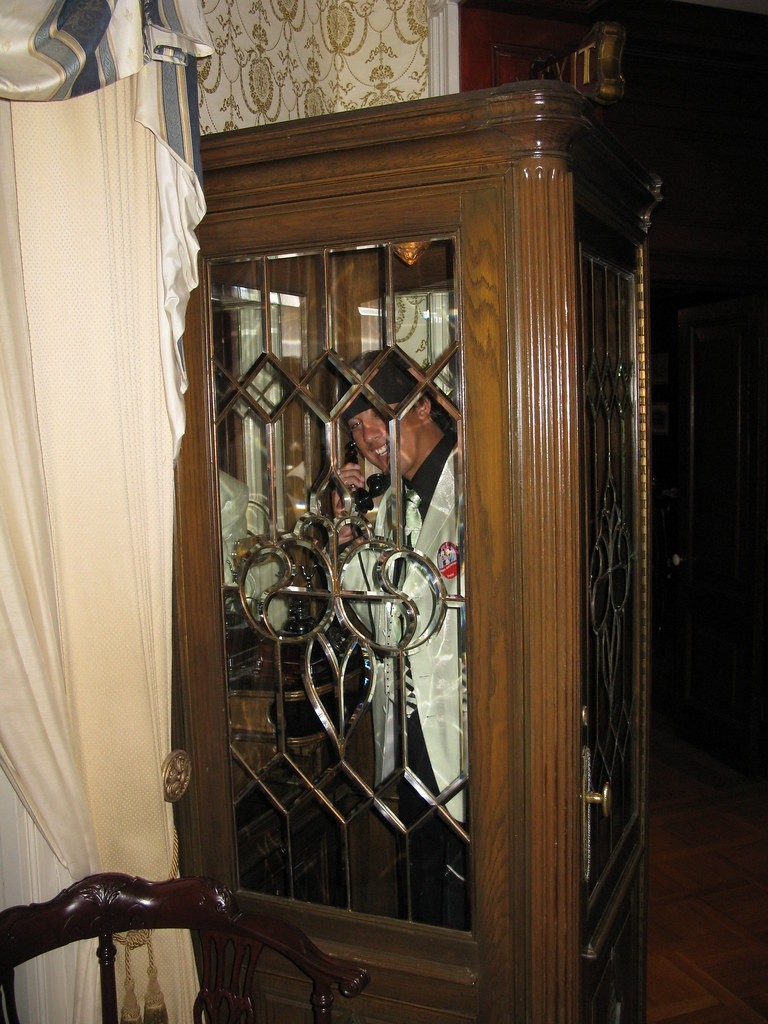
[226, 646, 370, 913]
[169, 80, 663, 1023]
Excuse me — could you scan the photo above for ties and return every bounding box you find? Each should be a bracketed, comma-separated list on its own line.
[403, 490, 423, 547]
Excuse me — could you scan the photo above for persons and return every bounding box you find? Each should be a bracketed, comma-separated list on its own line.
[332, 350, 467, 926]
[220, 471, 288, 631]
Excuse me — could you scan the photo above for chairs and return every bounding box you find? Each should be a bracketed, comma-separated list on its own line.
[0, 873, 371, 1024]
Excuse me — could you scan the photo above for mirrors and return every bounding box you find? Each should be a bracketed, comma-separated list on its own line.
[207, 283, 309, 648]
[359, 274, 452, 516]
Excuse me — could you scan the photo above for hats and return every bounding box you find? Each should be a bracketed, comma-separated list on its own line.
[342, 349, 425, 423]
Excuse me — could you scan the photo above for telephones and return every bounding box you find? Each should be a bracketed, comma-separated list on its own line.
[344, 440, 391, 513]
